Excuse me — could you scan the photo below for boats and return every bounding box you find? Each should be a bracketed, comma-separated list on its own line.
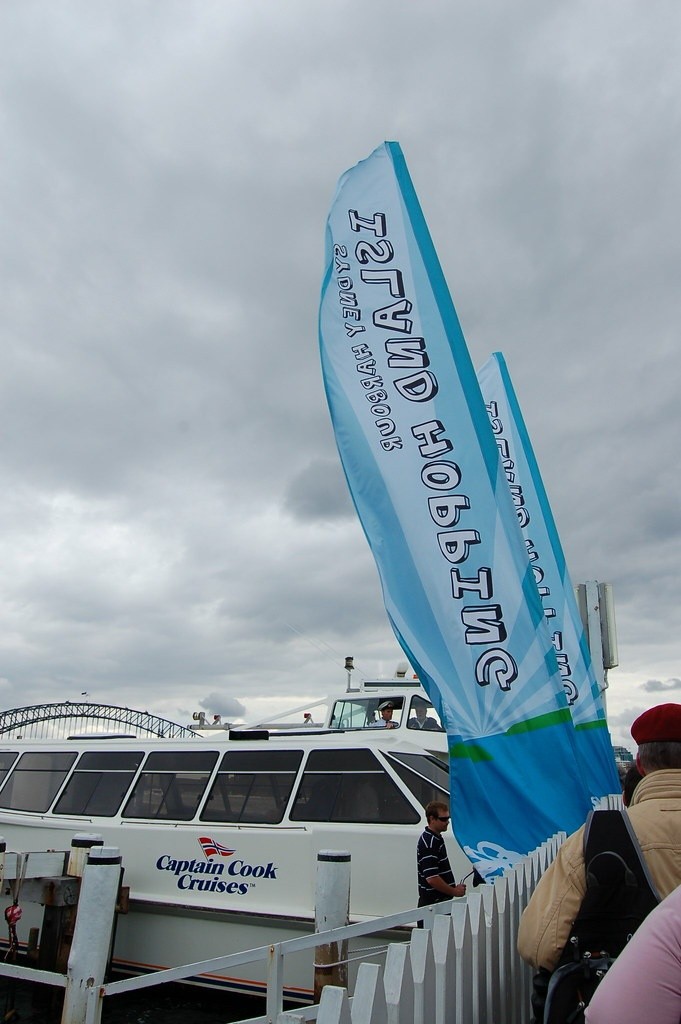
[0, 655, 474, 1005]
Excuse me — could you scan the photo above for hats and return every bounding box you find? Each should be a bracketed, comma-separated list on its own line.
[378, 700, 394, 711]
[631, 703, 681, 744]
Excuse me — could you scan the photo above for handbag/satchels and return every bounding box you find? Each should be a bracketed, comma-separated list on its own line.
[532, 810, 662, 1024]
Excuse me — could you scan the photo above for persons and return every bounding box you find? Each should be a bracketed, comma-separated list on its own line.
[516, 703, 681, 1024]
[416, 801, 466, 930]
[369, 701, 399, 729]
[407, 702, 443, 729]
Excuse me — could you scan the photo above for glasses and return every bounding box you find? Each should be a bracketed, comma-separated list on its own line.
[435, 816, 450, 821]
